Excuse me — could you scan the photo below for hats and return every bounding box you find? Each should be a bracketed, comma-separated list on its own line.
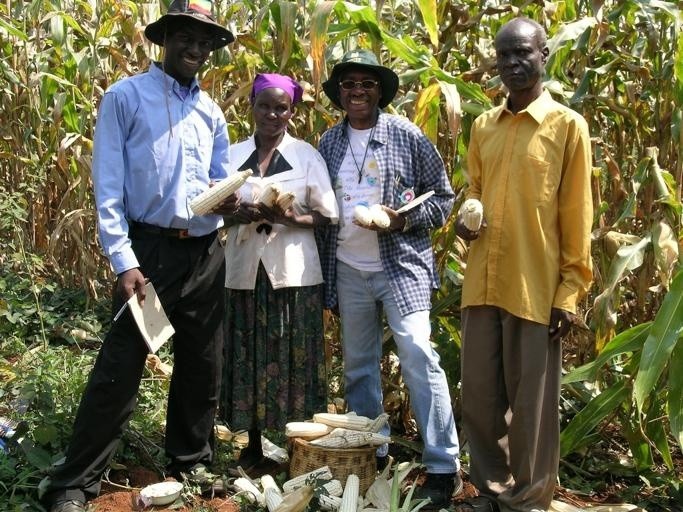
[145, 0, 235, 51]
[322, 50, 399, 110]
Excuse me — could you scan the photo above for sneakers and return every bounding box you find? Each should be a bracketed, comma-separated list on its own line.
[228, 447, 264, 477]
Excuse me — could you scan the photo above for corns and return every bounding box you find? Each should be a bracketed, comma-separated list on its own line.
[257, 185, 295, 212]
[461, 198, 484, 232]
[285, 411, 394, 448]
[353, 204, 391, 229]
[233, 465, 365, 512]
[189, 168, 253, 217]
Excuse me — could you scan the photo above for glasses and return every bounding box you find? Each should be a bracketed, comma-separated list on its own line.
[338, 80, 379, 90]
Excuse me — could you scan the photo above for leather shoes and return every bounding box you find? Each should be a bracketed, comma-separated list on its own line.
[418, 472, 464, 510]
[208, 479, 224, 494]
[51, 501, 84, 512]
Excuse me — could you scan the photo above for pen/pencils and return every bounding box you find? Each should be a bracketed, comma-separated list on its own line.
[113, 302, 127, 322]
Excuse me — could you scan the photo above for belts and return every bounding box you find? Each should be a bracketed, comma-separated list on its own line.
[139, 224, 192, 238]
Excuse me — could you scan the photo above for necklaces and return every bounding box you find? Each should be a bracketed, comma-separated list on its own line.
[348, 114, 375, 185]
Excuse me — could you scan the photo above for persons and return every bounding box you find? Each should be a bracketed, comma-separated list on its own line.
[445, 17, 594, 512]
[215, 75, 342, 479]
[313, 49, 464, 512]
[39, 0, 243, 512]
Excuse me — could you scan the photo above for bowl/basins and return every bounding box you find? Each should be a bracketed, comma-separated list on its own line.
[141, 481, 183, 506]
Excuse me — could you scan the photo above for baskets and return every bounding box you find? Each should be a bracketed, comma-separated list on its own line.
[288, 436, 377, 499]
